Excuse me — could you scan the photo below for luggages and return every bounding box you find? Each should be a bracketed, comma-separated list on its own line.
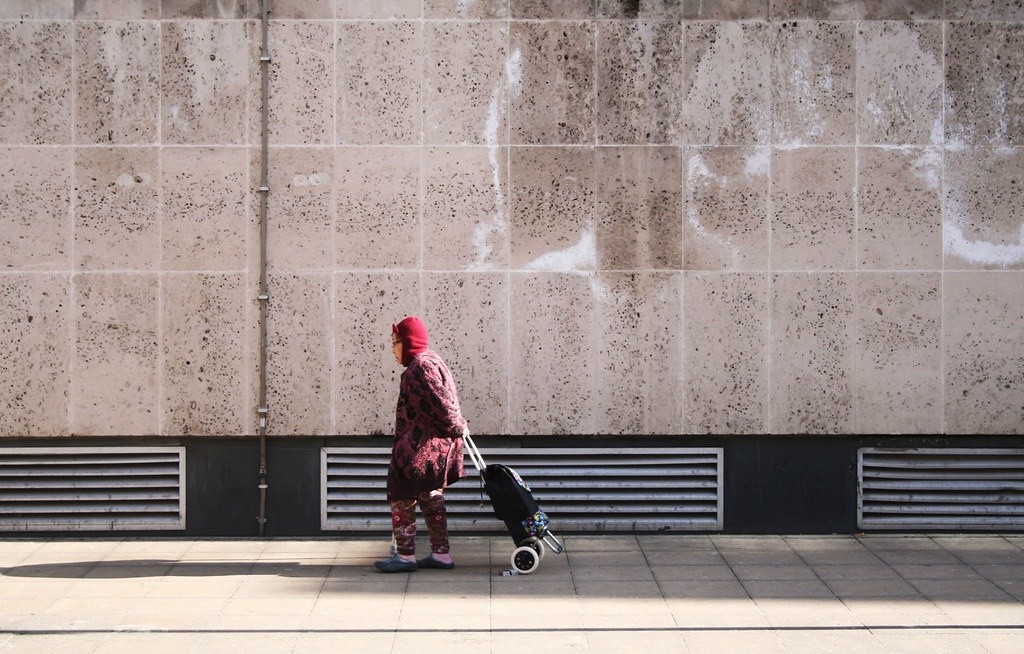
[462, 432, 563, 575]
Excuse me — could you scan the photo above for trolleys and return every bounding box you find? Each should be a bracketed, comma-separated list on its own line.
[461, 433, 563, 575]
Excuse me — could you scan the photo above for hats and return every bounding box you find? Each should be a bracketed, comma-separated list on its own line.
[392, 316, 428, 366]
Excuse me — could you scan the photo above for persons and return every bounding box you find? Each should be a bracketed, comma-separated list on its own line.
[373, 316, 470, 572]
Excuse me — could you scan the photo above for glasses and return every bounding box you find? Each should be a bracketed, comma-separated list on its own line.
[391, 340, 402, 347]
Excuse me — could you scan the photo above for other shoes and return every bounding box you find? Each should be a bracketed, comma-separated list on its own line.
[374, 553, 418, 572]
[416, 552, 454, 569]
